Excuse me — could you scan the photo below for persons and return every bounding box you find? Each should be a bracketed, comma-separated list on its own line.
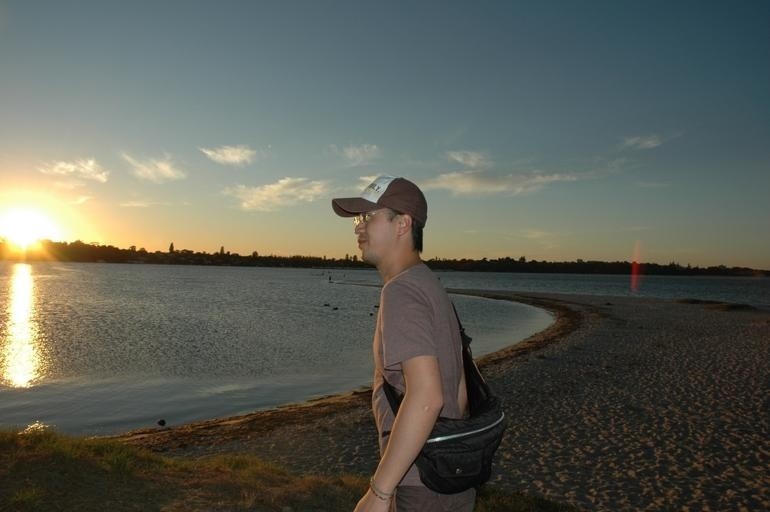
[332, 173, 477, 512]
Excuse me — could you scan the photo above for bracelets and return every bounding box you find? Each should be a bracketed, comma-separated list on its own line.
[368, 476, 397, 501]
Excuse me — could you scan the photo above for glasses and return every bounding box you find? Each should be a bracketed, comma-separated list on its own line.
[353, 210, 388, 226]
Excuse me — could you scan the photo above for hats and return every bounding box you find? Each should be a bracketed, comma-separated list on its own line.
[332, 175, 427, 225]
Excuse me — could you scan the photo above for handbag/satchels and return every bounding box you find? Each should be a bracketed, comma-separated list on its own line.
[383, 312, 507, 495]
